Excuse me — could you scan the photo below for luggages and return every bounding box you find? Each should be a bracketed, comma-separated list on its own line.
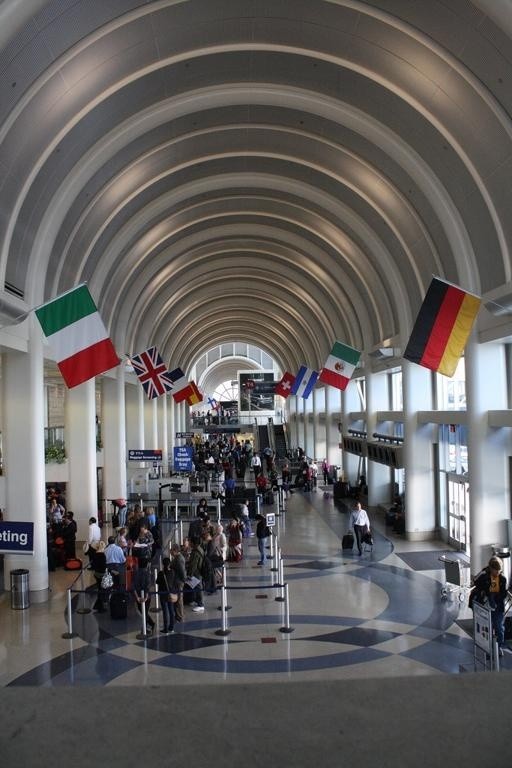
[342, 529, 354, 551]
[109, 591, 129, 620]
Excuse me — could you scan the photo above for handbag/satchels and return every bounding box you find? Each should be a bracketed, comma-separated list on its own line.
[168, 593, 179, 603]
[101, 573, 114, 589]
[209, 554, 224, 569]
[365, 532, 374, 545]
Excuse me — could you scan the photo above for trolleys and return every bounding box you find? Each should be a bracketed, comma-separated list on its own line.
[437, 555, 475, 603]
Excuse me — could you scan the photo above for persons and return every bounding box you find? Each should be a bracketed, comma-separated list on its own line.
[470, 555, 508, 662]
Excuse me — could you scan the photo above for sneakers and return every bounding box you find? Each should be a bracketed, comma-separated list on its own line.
[192, 606, 205, 612]
[257, 561, 264, 565]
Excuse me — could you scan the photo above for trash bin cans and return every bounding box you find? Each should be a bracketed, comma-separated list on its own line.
[137, 499, 143, 512]
[159, 466, 162, 478]
[9, 569, 30, 610]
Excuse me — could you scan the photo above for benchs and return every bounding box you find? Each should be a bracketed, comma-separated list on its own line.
[376, 500, 403, 523]
[350, 485, 368, 504]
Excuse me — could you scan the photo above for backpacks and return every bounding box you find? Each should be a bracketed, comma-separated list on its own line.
[192, 548, 213, 579]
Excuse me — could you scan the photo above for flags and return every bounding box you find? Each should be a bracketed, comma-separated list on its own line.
[275, 369, 296, 398]
[32, 282, 120, 390]
[130, 346, 221, 411]
[403, 275, 484, 378]
[319, 342, 360, 391]
[289, 366, 318, 399]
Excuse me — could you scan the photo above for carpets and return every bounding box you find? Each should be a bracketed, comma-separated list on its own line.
[395, 549, 470, 571]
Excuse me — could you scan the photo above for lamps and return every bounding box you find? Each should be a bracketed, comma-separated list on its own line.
[483, 293, 512, 317]
[367, 347, 394, 360]
